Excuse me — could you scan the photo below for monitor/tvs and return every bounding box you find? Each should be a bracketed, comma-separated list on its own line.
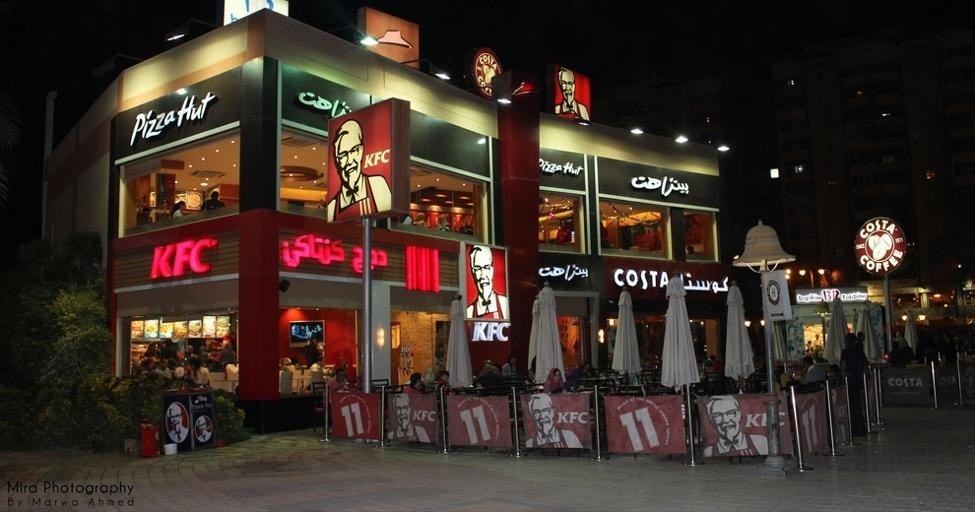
[289, 320, 325, 348]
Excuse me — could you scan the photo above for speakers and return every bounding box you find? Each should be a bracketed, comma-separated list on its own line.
[279, 279, 291, 292]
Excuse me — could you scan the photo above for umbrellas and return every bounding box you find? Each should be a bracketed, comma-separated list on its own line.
[658, 270, 702, 466]
[442, 293, 475, 391]
[722, 279, 756, 394]
[905, 310, 919, 356]
[534, 281, 568, 386]
[856, 309, 882, 431]
[611, 285, 645, 374]
[822, 293, 851, 367]
[526, 293, 540, 372]
[772, 319, 792, 376]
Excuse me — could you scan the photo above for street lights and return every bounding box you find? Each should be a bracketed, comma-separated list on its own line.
[795, 266, 826, 290]
[731, 219, 798, 466]
[901, 310, 928, 361]
[811, 297, 833, 368]
[742, 318, 768, 380]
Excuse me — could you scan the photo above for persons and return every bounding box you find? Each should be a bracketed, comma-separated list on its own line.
[201, 190, 225, 211]
[709, 354, 716, 362]
[857, 331, 865, 349]
[501, 355, 519, 378]
[137, 334, 238, 387]
[840, 331, 869, 437]
[604, 353, 613, 370]
[466, 242, 507, 321]
[543, 367, 565, 394]
[699, 354, 713, 368]
[639, 353, 653, 383]
[401, 377, 425, 394]
[702, 395, 770, 458]
[800, 355, 825, 385]
[325, 116, 393, 224]
[438, 370, 452, 395]
[322, 368, 351, 409]
[776, 363, 791, 389]
[812, 333, 822, 349]
[787, 324, 799, 350]
[415, 211, 425, 223]
[478, 365, 505, 390]
[387, 392, 431, 444]
[831, 389, 847, 443]
[553, 65, 590, 122]
[889, 340, 905, 366]
[297, 336, 325, 369]
[196, 416, 211, 442]
[171, 200, 186, 219]
[437, 215, 450, 232]
[527, 355, 537, 380]
[525, 392, 584, 450]
[166, 405, 188, 442]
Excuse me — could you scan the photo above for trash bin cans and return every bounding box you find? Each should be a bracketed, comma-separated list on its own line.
[162, 389, 215, 451]
[139, 423, 159, 456]
